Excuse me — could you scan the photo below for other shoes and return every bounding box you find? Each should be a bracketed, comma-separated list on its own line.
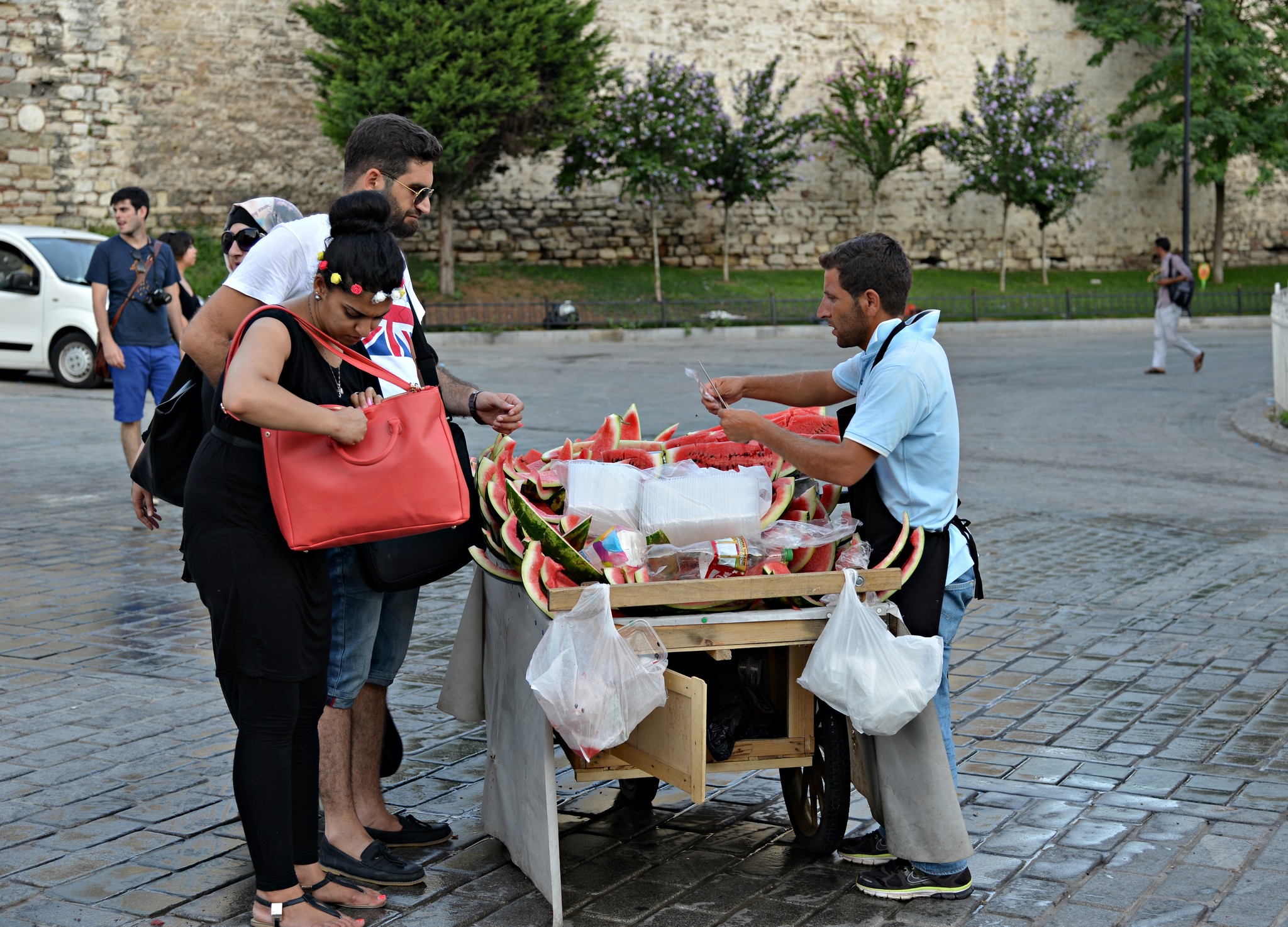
[364, 813, 453, 847]
[131, 497, 158, 506]
[319, 834, 427, 886]
[1195, 352, 1204, 371]
[1144, 367, 1166, 374]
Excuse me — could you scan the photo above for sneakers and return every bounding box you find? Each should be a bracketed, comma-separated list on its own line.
[856, 857, 974, 900]
[838, 829, 898, 864]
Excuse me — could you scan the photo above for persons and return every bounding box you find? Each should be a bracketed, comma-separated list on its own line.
[85, 186, 185, 504]
[1146, 237, 1204, 376]
[157, 231, 202, 354]
[133, 196, 405, 780]
[179, 115, 523, 886]
[701, 232, 984, 899]
[180, 190, 405, 927]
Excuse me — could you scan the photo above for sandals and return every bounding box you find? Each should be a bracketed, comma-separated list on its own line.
[299, 870, 387, 909]
[251, 890, 364, 927]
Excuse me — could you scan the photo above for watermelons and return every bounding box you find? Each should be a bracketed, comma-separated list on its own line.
[602, 401, 841, 482]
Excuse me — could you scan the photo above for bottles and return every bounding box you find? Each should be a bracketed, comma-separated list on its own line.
[639, 535, 793, 583]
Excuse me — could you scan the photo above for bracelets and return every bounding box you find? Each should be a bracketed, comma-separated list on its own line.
[469, 391, 487, 425]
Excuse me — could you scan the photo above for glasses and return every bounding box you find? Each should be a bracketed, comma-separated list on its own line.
[221, 228, 267, 254]
[379, 170, 435, 205]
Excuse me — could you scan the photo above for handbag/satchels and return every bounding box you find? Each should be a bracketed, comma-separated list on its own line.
[93, 332, 111, 379]
[527, 584, 666, 760]
[129, 380, 206, 508]
[797, 567, 945, 737]
[1170, 271, 1194, 309]
[353, 419, 490, 595]
[219, 304, 471, 549]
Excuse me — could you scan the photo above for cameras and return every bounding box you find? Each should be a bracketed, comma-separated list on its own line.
[143, 287, 173, 313]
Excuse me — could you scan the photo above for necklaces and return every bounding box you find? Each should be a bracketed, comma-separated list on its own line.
[307, 295, 344, 398]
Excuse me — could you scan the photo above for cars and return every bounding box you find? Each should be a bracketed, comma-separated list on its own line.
[0, 224, 110, 389]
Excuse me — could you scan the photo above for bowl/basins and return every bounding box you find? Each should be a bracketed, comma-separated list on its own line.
[565, 460, 761, 547]
